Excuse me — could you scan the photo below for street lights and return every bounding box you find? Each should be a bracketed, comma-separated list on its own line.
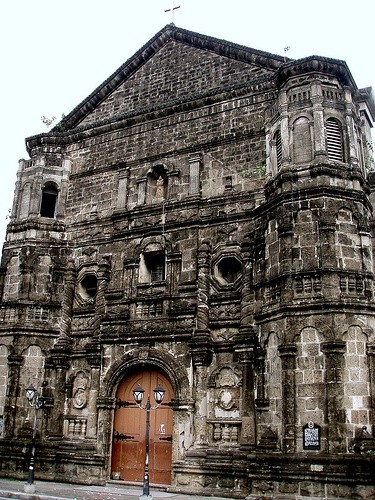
[24, 383, 55, 484]
[132, 383, 166, 496]
